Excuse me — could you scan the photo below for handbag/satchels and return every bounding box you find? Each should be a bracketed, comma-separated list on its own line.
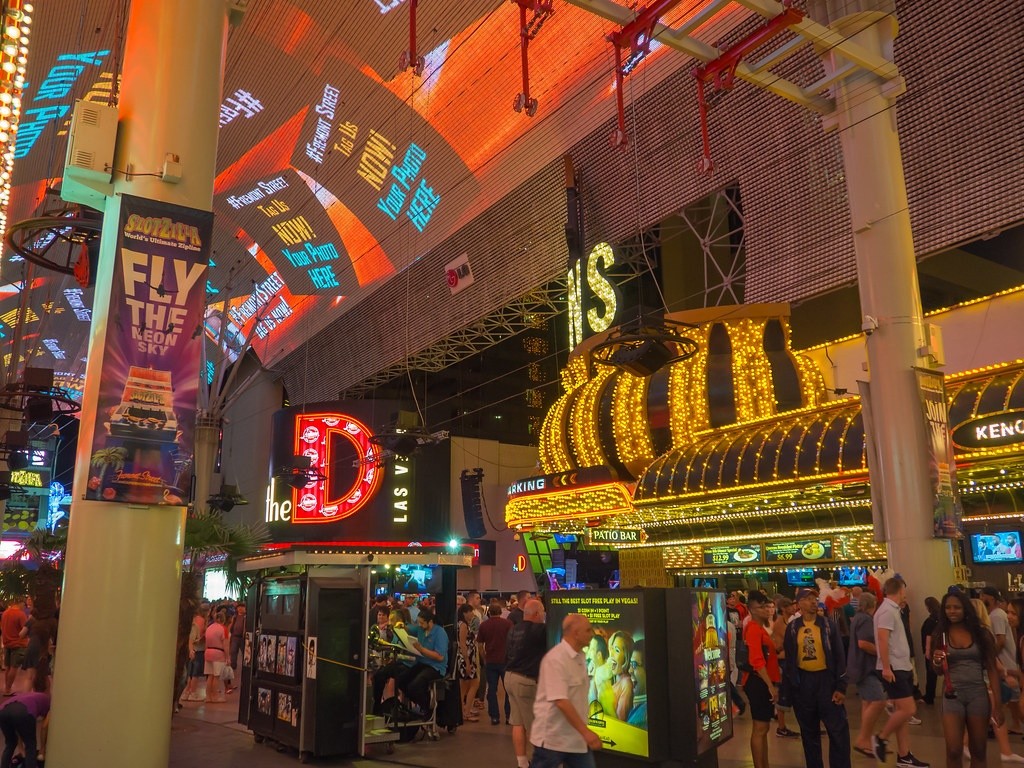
[221, 662, 235, 681]
[735, 643, 768, 672]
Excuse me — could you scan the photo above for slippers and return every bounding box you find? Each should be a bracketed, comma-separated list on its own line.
[852, 746, 875, 759]
[3, 692, 17, 697]
[886, 749, 893, 756]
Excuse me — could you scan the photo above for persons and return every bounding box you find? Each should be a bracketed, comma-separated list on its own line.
[181, 603, 246, 703]
[456, 590, 530, 725]
[0, 594, 62, 768]
[369, 595, 450, 728]
[529, 614, 603, 768]
[584, 630, 647, 731]
[503, 600, 547, 768]
[724, 577, 1024, 768]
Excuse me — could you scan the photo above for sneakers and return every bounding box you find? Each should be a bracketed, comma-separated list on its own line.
[896, 750, 930, 768]
[777, 726, 801, 739]
[871, 734, 888, 764]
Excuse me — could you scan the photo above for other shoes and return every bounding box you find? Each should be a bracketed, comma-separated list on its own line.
[490, 717, 501, 725]
[962, 748, 971, 761]
[188, 692, 205, 701]
[885, 704, 894, 716]
[212, 698, 227, 703]
[906, 717, 922, 727]
[999, 753, 1024, 766]
[180, 692, 188, 701]
[463, 715, 479, 723]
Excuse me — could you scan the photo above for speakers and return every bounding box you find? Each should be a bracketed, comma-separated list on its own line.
[288, 475, 308, 489]
[5, 431, 27, 450]
[610, 341, 672, 379]
[292, 456, 311, 469]
[383, 434, 417, 457]
[618, 306, 664, 335]
[391, 410, 419, 430]
[24, 367, 54, 393]
[461, 475, 486, 537]
[0, 454, 29, 500]
[26, 397, 53, 422]
[74, 239, 101, 289]
[211, 485, 236, 512]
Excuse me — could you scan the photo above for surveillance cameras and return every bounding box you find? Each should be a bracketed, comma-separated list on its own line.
[514, 534, 520, 541]
[862, 320, 875, 335]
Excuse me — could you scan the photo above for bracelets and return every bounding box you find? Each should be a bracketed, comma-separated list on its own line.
[933, 660, 941, 666]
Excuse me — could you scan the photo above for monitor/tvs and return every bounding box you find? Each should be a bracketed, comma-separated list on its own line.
[786, 568, 815, 587]
[694, 578, 718, 589]
[968, 530, 1024, 564]
[839, 567, 868, 586]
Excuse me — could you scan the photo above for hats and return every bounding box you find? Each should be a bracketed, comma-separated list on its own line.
[404, 594, 415, 600]
[981, 585, 1006, 602]
[797, 587, 820, 601]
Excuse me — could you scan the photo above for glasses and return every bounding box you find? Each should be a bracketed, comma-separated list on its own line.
[754, 605, 770, 609]
[802, 597, 819, 601]
